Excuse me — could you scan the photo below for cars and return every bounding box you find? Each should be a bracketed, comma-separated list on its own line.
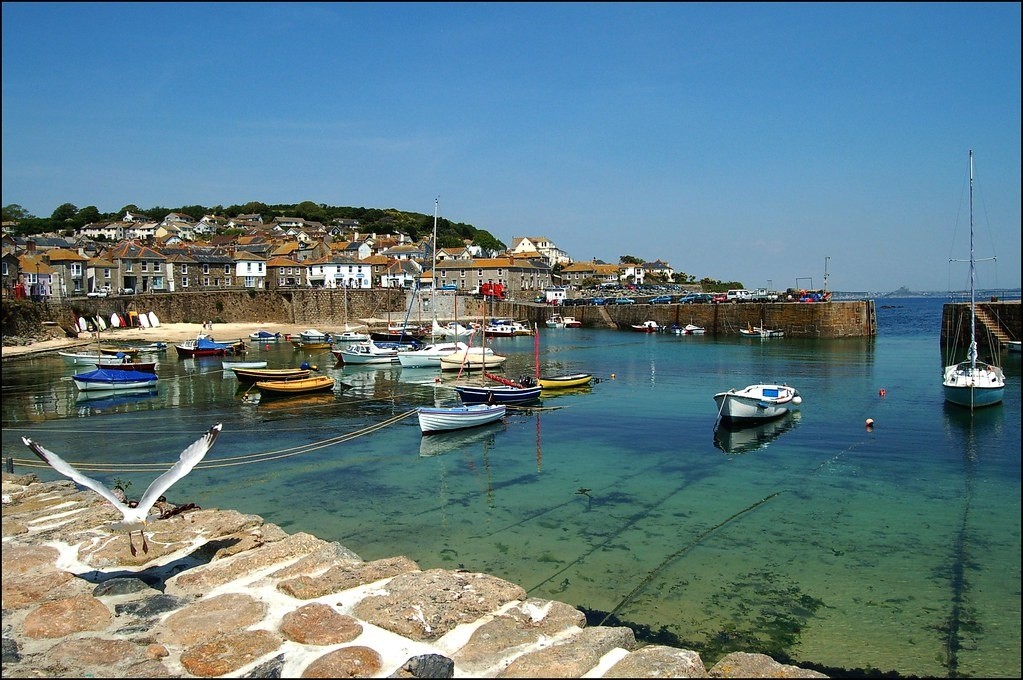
[679, 293, 697, 304]
[697, 288, 779, 304]
[534, 296, 546, 303]
[563, 297, 635, 305]
[545, 283, 680, 290]
[648, 295, 672, 304]
[100, 285, 113, 295]
[119, 288, 134, 295]
[87, 290, 107, 299]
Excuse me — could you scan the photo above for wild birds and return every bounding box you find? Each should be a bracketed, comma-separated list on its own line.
[22, 422, 223, 555]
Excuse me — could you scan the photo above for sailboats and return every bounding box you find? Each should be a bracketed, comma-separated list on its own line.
[941, 149, 1006, 414]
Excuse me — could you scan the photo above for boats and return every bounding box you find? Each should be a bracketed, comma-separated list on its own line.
[58, 198, 596, 435]
[712, 381, 803, 429]
[713, 410, 802, 454]
[545, 311, 582, 329]
[738, 318, 785, 338]
[420, 420, 506, 457]
[670, 317, 707, 335]
[631, 319, 667, 334]
